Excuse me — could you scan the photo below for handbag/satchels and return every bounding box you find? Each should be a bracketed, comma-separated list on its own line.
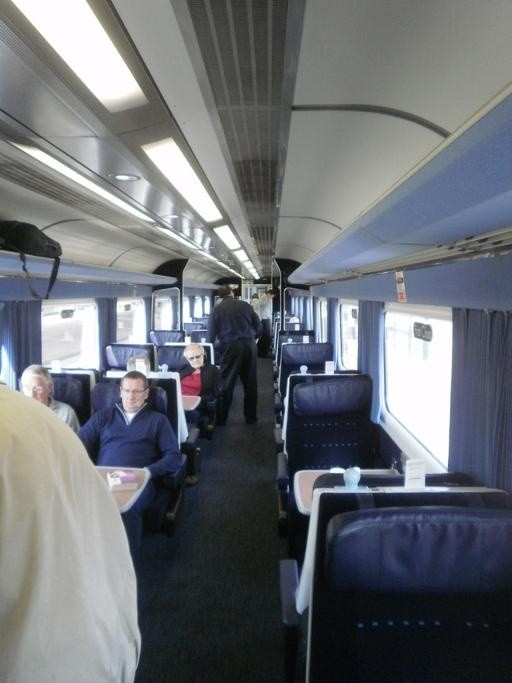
[0, 221, 63, 258]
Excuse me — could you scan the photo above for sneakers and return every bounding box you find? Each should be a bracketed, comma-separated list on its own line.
[246, 417, 258, 424]
[184, 476, 199, 487]
[216, 417, 227, 426]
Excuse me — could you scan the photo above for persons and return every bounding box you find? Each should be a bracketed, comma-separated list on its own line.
[250, 293, 263, 348]
[206, 286, 262, 426]
[79, 370, 181, 571]
[260, 290, 276, 348]
[20, 366, 81, 438]
[0, 369, 143, 683]
[176, 344, 221, 485]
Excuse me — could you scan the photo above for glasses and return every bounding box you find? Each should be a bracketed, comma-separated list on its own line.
[188, 355, 202, 361]
